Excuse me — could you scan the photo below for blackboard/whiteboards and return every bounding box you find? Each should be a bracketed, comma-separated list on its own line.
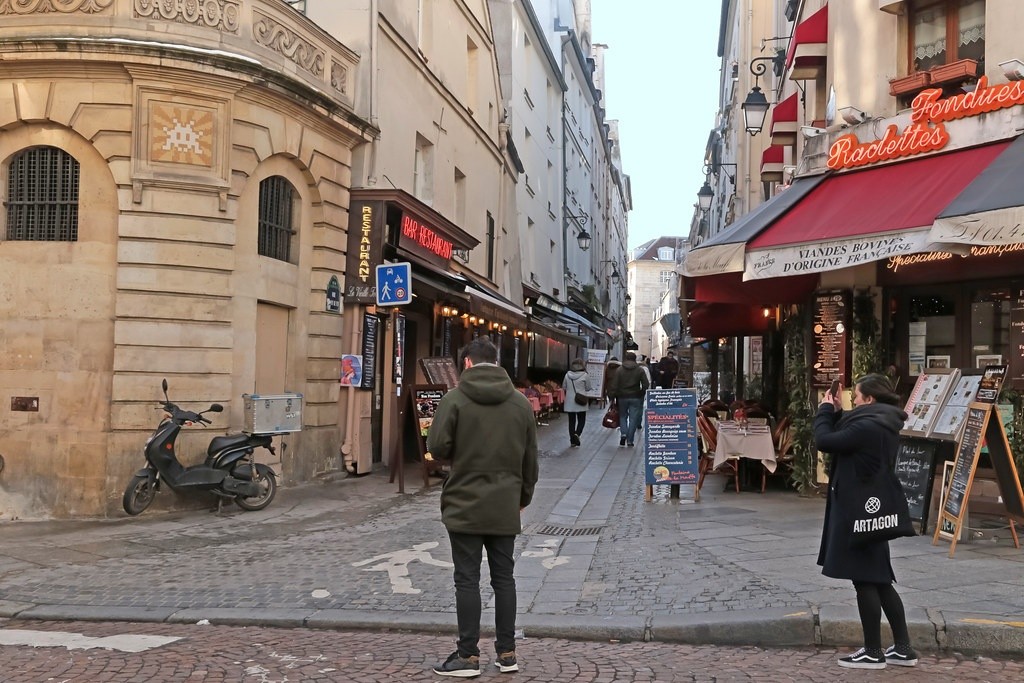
[937, 460, 962, 540]
[894, 437, 938, 522]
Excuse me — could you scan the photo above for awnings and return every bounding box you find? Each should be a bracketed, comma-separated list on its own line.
[760, 146, 784, 182]
[785, 6, 828, 81]
[926, 133, 1024, 249]
[743, 139, 1016, 284]
[462, 270, 587, 348]
[769, 90, 798, 146]
[386, 246, 528, 331]
[524, 282, 639, 350]
[672, 170, 835, 277]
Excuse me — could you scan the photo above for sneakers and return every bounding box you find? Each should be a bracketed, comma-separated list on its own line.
[884, 644, 918, 667]
[837, 647, 887, 669]
[495, 650, 519, 672]
[432, 649, 480, 677]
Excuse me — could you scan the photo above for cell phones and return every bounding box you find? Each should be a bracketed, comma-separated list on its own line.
[829, 380, 840, 400]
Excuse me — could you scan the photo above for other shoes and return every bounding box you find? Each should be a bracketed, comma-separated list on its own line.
[571, 442, 576, 447]
[620, 436, 626, 445]
[573, 434, 581, 446]
[628, 444, 634, 446]
[637, 426, 642, 429]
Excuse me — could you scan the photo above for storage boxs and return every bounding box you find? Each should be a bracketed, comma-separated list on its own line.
[242, 393, 303, 435]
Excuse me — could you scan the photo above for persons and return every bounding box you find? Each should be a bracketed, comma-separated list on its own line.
[613, 352, 648, 446]
[635, 351, 679, 432]
[814, 374, 919, 670]
[602, 357, 622, 409]
[425, 337, 539, 677]
[562, 358, 592, 447]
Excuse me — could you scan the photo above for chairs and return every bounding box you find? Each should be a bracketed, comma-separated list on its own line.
[527, 380, 562, 425]
[697, 400, 796, 493]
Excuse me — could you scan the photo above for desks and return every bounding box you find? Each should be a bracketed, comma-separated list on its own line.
[530, 393, 553, 423]
[528, 398, 540, 427]
[541, 388, 566, 414]
[709, 416, 778, 493]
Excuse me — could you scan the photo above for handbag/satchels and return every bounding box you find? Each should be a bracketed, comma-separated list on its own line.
[847, 423, 919, 551]
[574, 393, 587, 406]
[603, 400, 619, 428]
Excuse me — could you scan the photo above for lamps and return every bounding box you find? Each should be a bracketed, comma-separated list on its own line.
[777, 184, 791, 191]
[838, 106, 863, 125]
[560, 215, 591, 252]
[799, 125, 827, 138]
[999, 58, 1024, 81]
[762, 303, 775, 319]
[600, 255, 620, 286]
[649, 334, 653, 342]
[625, 294, 631, 304]
[741, 55, 786, 137]
[783, 165, 797, 175]
[697, 162, 737, 214]
[731, 61, 738, 82]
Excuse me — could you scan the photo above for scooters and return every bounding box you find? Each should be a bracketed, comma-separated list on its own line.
[122, 378, 291, 517]
